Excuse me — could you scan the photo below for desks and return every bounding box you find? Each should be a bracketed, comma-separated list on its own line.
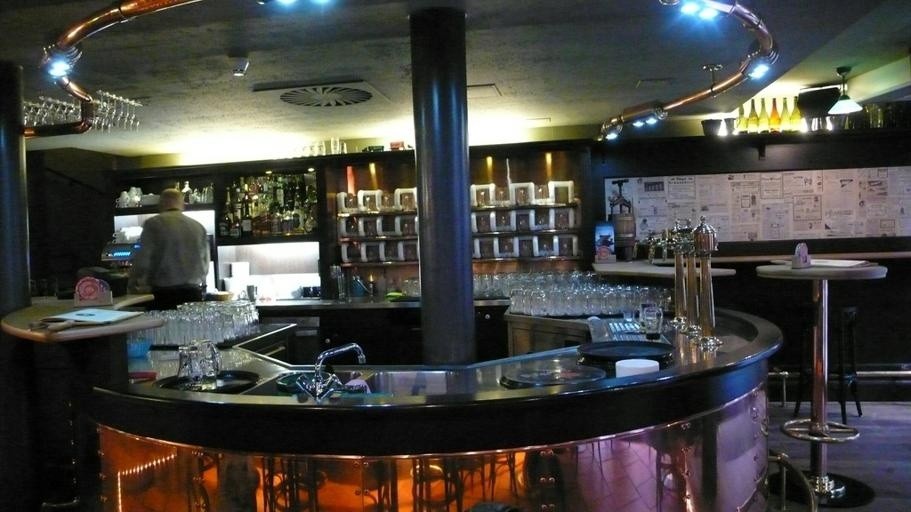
[1, 294, 155, 511]
[591, 249, 911, 407]
[756, 264, 889, 509]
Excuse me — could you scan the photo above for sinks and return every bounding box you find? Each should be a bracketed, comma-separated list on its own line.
[353, 368, 469, 397]
[240, 370, 363, 397]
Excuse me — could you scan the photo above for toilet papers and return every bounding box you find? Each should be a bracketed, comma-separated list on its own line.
[615, 357, 660, 378]
[232, 261, 249, 302]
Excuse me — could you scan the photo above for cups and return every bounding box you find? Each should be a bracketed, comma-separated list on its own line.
[402, 277, 420, 297]
[115, 187, 143, 208]
[633, 304, 664, 340]
[472, 270, 674, 317]
[247, 284, 258, 302]
[128, 301, 261, 346]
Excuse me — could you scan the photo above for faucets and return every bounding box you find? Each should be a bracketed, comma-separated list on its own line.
[295, 343, 366, 405]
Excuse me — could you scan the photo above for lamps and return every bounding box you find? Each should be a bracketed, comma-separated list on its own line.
[826, 67, 863, 116]
[41, 0, 779, 143]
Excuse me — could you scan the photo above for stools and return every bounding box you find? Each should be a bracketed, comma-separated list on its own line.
[793, 295, 863, 424]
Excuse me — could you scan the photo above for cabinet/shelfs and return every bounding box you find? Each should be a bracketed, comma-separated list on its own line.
[96, 159, 340, 298]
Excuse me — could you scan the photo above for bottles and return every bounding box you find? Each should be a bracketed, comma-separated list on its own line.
[176, 338, 221, 384]
[175, 181, 214, 204]
[351, 267, 376, 297]
[302, 287, 321, 297]
[736, 96, 802, 135]
[219, 172, 318, 236]
[330, 265, 346, 299]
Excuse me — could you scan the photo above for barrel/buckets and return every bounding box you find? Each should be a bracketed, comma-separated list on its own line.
[614, 215, 636, 260]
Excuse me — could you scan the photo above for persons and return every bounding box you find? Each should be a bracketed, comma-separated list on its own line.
[128, 188, 210, 311]
